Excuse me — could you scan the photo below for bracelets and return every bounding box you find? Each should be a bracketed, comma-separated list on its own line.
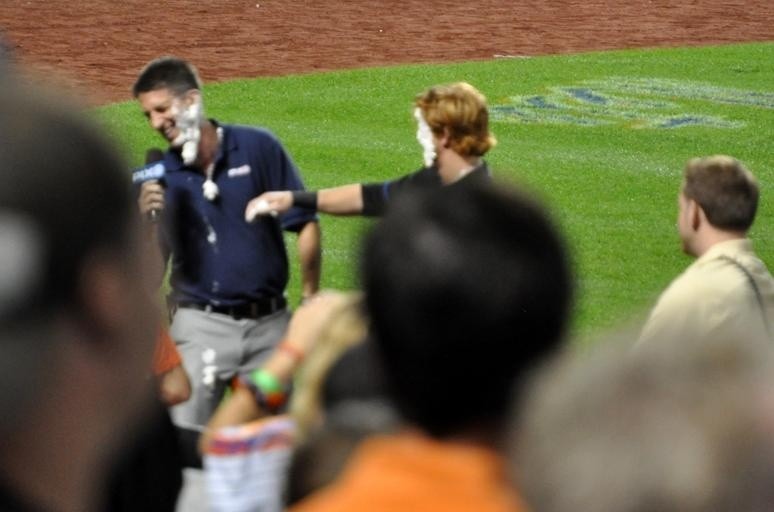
[295, 190, 319, 209]
[245, 370, 289, 411]
[279, 343, 308, 364]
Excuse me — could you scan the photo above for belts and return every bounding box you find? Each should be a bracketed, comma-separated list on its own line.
[176, 296, 286, 319]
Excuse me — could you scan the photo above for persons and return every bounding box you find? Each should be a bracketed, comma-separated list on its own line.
[628, 156, 773, 375]
[510, 324, 774, 511]
[245, 83, 495, 224]
[287, 180, 574, 512]
[132, 61, 322, 461]
[1, 55, 160, 511]
[200, 287, 371, 453]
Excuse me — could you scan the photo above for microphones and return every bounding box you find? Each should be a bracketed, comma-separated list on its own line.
[140, 147, 165, 223]
